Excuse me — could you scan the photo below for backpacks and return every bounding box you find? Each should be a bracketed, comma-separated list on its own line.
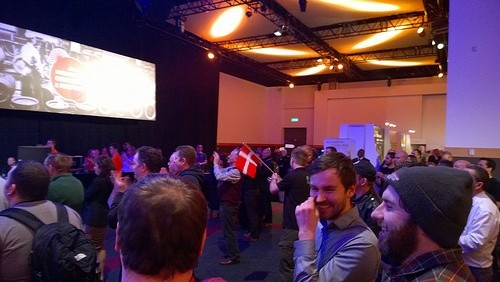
[0, 201, 102, 282]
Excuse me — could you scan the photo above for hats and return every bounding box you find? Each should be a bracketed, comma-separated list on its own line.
[385, 167, 473, 248]
[355, 161, 377, 179]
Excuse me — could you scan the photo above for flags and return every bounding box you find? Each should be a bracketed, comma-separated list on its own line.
[234, 145, 261, 178]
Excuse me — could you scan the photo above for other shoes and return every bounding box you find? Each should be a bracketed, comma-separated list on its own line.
[243, 236, 258, 241]
[220, 258, 236, 265]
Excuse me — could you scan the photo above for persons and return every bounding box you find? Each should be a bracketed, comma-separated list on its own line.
[0, 140, 500, 282]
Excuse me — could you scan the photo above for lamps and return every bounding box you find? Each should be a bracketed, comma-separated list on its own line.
[208, 44, 215, 59]
[418, 27, 426, 38]
[274, 28, 282, 37]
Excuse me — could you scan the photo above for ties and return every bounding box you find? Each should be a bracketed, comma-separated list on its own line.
[315, 219, 335, 258]
